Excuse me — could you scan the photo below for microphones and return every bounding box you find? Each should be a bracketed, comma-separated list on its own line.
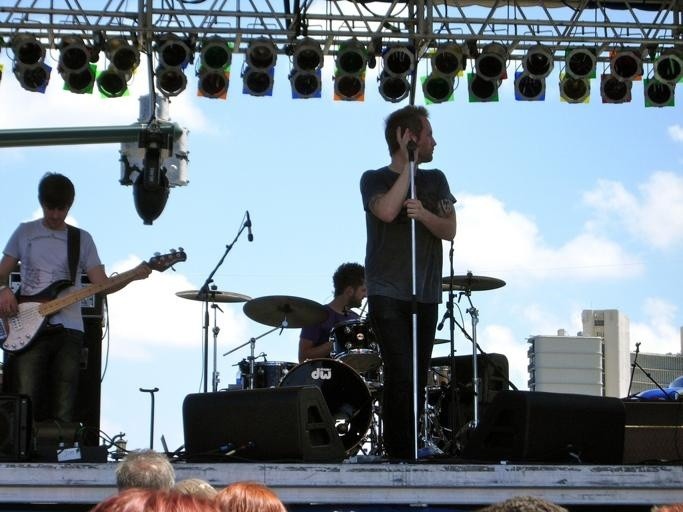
[405, 139, 418, 153]
[246, 211, 254, 241]
[437, 308, 451, 331]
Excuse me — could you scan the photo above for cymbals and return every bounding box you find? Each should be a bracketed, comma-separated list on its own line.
[433, 338, 451, 346]
[177, 291, 252, 304]
[442, 273, 504, 290]
[243, 296, 329, 329]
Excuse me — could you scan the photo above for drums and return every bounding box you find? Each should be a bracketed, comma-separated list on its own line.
[278, 356, 374, 455]
[240, 358, 298, 389]
[329, 318, 384, 374]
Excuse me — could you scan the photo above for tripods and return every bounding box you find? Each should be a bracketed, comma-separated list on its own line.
[417, 387, 449, 454]
[345, 366, 384, 457]
[442, 295, 479, 452]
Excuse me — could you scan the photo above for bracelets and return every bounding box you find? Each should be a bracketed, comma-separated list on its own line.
[0, 284, 7, 290]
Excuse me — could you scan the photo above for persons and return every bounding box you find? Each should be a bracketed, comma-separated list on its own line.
[0, 174, 152, 462]
[298, 263, 368, 363]
[359, 105, 456, 463]
[480, 496, 567, 512]
[92, 452, 287, 512]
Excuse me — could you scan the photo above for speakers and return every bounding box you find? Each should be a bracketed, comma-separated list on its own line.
[182, 385, 347, 463]
[461, 390, 628, 464]
[0, 395, 32, 462]
[3, 324, 102, 447]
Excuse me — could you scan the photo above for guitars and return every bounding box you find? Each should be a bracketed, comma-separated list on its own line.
[2, 248, 187, 353]
